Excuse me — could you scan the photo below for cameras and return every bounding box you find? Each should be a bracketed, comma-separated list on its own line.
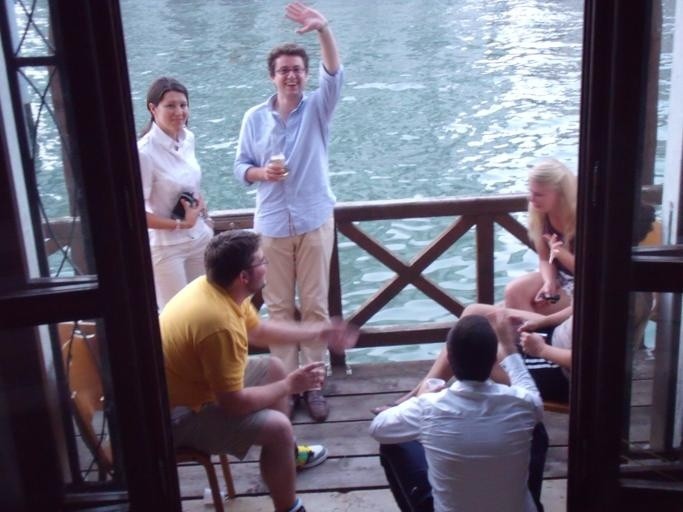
[171, 192, 194, 220]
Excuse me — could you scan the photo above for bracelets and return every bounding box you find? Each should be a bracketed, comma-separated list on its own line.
[317, 22, 328, 34]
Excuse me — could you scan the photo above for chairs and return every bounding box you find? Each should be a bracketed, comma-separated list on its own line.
[543, 218, 663, 416]
[54, 335, 234, 512]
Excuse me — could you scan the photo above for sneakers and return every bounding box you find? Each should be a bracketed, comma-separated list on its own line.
[294, 444, 328, 470]
[303, 389, 329, 420]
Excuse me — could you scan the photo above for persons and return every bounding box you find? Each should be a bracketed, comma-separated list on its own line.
[133, 77, 216, 312]
[156, 227, 363, 511]
[499, 157, 580, 344]
[366, 298, 551, 512]
[367, 197, 656, 417]
[230, 1, 345, 427]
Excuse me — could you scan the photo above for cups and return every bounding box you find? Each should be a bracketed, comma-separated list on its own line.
[203, 487, 225, 505]
[270, 153, 286, 182]
[425, 376, 446, 394]
[298, 362, 325, 391]
